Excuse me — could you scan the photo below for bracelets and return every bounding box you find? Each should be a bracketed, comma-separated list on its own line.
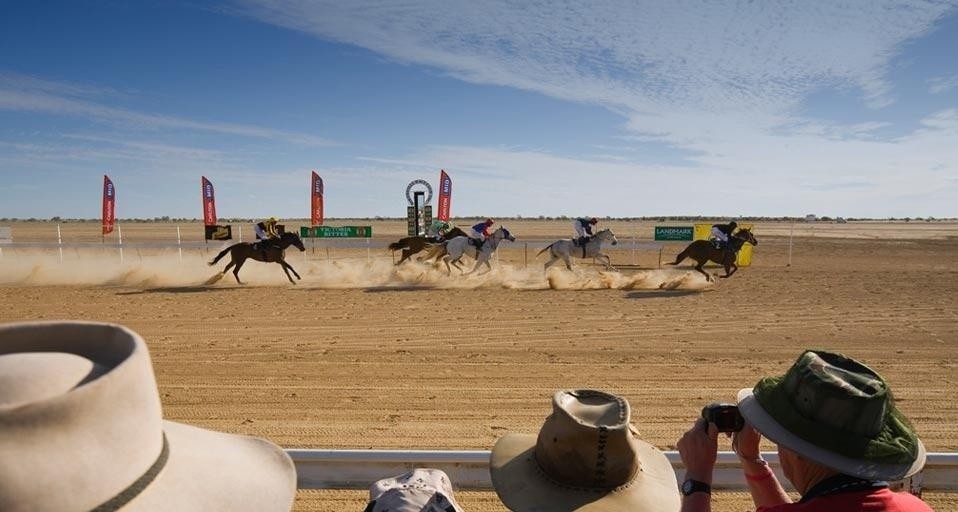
[744, 471, 773, 483]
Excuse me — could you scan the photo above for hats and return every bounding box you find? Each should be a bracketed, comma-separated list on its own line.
[734, 345, 928, 482]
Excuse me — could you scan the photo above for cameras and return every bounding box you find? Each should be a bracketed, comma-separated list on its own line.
[701, 403, 744, 432]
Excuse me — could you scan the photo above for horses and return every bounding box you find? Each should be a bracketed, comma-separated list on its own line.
[208, 230, 305, 285]
[663, 227, 758, 281]
[421, 224, 516, 276]
[536, 228, 620, 275]
[387, 226, 468, 266]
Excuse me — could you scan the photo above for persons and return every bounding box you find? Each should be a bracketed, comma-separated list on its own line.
[250, 216, 281, 251]
[674, 347, 934, 512]
[467, 218, 494, 245]
[0, 318, 300, 512]
[571, 217, 598, 245]
[426, 220, 449, 241]
[710, 221, 737, 250]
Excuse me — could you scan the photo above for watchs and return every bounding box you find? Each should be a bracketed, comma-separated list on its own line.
[680, 479, 711, 497]
